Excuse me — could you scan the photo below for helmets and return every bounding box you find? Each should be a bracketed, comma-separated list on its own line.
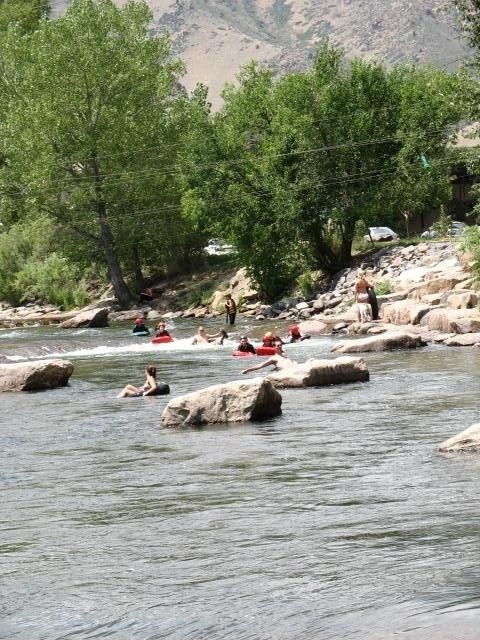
[240, 335, 247, 341]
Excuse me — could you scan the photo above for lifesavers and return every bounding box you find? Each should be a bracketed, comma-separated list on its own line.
[232, 351, 253, 357]
[131, 331, 150, 336]
[152, 336, 174, 344]
[256, 347, 282, 355]
[128, 383, 170, 396]
[367, 286, 378, 320]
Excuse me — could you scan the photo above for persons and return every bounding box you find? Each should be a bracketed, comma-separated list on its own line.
[224, 293, 237, 325]
[191, 325, 229, 345]
[238, 336, 256, 354]
[286, 325, 303, 341]
[155, 323, 169, 338]
[240, 338, 295, 376]
[355, 272, 374, 322]
[133, 318, 151, 334]
[139, 287, 154, 305]
[119, 365, 157, 398]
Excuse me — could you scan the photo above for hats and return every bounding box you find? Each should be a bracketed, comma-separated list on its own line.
[262, 331, 275, 342]
[291, 325, 299, 335]
[134, 318, 166, 329]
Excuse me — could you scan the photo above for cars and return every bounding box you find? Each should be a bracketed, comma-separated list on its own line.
[421, 221, 470, 237]
[204, 237, 233, 256]
[363, 227, 400, 242]
